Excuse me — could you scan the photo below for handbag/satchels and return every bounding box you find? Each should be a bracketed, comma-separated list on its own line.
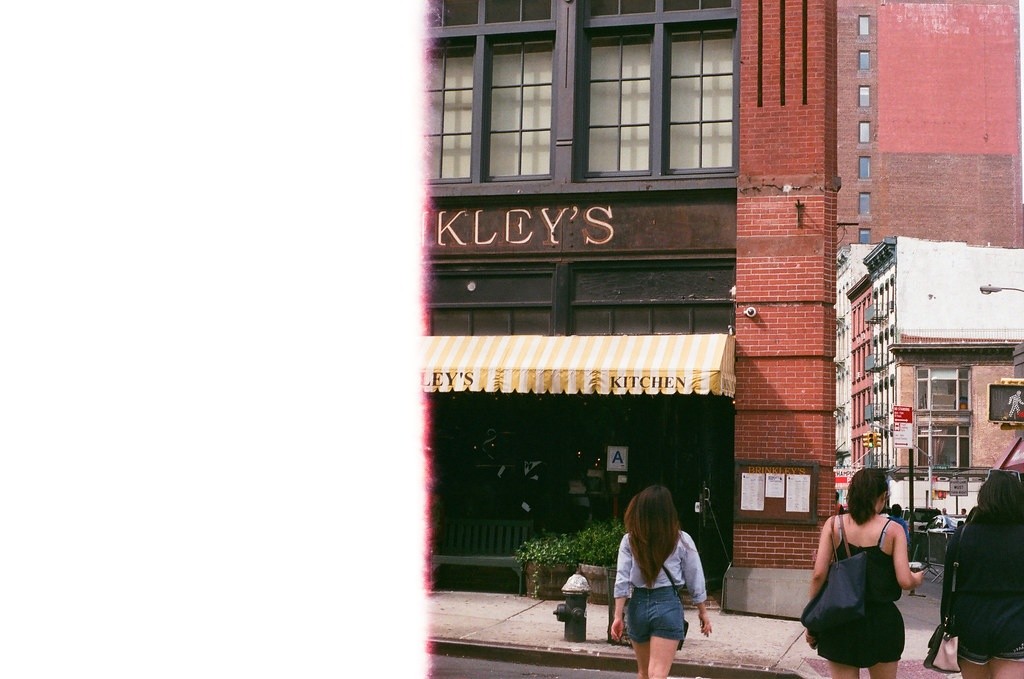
[801, 514, 867, 635]
[677, 620, 689, 651]
[923, 615, 961, 674]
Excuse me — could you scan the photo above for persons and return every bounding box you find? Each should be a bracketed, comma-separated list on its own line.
[880, 507, 890, 517]
[940, 469, 1024, 679]
[800, 467, 924, 679]
[888, 505, 910, 542]
[836, 492, 845, 514]
[611, 486, 713, 679]
[942, 508, 948, 515]
[961, 509, 966, 515]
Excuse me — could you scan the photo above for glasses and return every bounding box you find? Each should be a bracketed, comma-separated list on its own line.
[988, 469, 1021, 482]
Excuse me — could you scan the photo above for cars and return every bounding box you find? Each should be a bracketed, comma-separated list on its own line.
[918, 514, 968, 548]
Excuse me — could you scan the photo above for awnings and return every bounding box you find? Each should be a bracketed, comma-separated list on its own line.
[423, 332, 737, 398]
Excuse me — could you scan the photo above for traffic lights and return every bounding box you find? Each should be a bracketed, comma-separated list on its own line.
[988, 385, 1024, 423]
[862, 431, 874, 447]
[874, 433, 882, 448]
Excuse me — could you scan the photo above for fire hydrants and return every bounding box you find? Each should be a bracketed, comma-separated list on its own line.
[552, 571, 590, 642]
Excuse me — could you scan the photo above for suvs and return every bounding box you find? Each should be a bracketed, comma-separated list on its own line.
[900, 509, 942, 536]
